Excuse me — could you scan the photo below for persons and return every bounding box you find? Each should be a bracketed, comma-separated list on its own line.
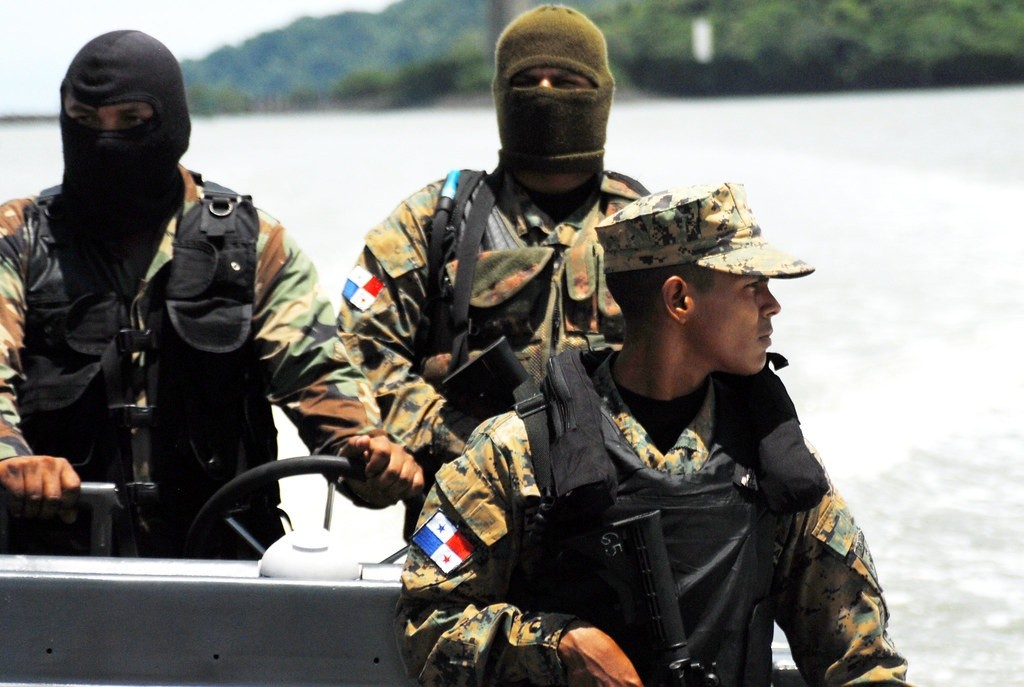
[334, 3, 651, 547]
[390, 176, 909, 687]
[0, 30, 424, 560]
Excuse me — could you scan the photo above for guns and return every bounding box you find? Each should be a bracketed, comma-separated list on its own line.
[600, 507, 721, 687]
[440, 335, 531, 419]
[124, 474, 182, 557]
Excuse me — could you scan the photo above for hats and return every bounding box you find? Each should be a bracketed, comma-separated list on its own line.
[595, 181, 816, 278]
